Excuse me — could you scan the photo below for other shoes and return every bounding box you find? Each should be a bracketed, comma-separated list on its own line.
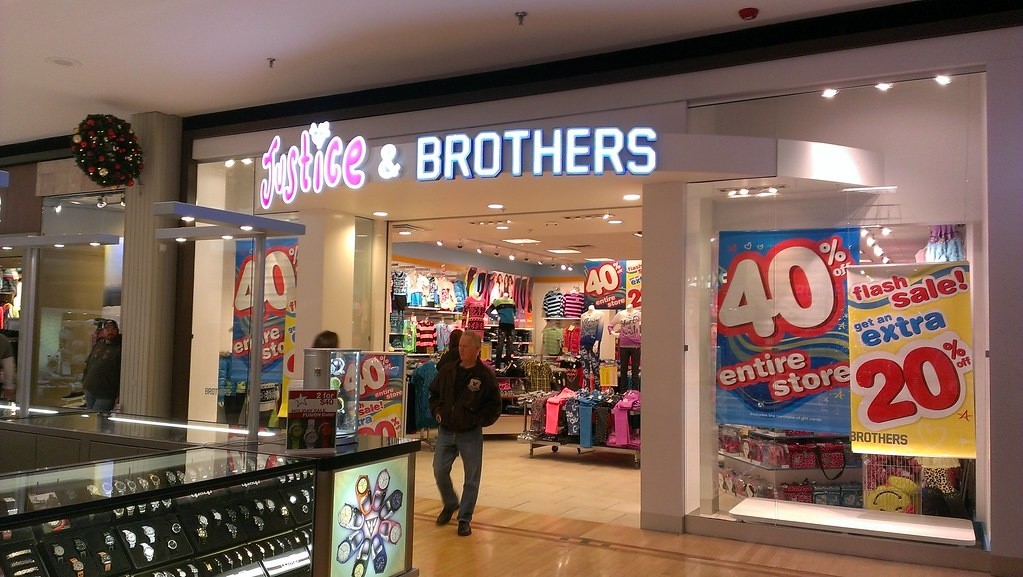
[61, 391, 86, 401]
[435, 504, 459, 523]
[458, 520, 471, 536]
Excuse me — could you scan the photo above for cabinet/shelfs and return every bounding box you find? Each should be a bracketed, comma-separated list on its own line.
[518, 401, 641, 468]
[543, 318, 581, 358]
[716, 424, 862, 507]
[391, 305, 463, 357]
[483, 325, 536, 360]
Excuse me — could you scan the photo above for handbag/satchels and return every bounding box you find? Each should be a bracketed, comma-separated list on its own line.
[780, 483, 813, 503]
[815, 442, 845, 479]
[787, 443, 815, 469]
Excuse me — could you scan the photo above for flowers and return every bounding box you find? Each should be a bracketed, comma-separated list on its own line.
[74, 113, 145, 188]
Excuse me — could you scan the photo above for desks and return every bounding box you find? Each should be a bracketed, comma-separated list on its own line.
[482, 395, 527, 438]
[728, 496, 977, 549]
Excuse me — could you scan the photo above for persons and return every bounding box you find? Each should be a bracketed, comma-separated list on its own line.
[0, 334, 16, 400]
[311, 331, 339, 349]
[460, 290, 518, 371]
[435, 329, 465, 370]
[428, 329, 502, 537]
[81, 319, 123, 412]
[580, 304, 642, 393]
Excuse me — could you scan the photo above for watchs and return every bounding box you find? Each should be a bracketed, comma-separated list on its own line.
[3, 497, 18, 515]
[336, 468, 404, 576]
[40, 451, 314, 577]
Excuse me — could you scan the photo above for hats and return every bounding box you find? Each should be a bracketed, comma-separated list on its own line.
[102, 318, 118, 330]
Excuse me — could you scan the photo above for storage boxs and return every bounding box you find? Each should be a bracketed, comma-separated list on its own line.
[860, 454, 924, 516]
[303, 347, 363, 444]
[720, 424, 862, 508]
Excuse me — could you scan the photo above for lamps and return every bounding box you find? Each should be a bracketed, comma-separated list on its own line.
[476, 248, 483, 253]
[633, 231, 643, 238]
[56, 198, 61, 212]
[456, 239, 464, 250]
[494, 246, 500, 257]
[120, 194, 125, 207]
[538, 261, 543, 266]
[524, 251, 530, 262]
[549, 258, 556, 268]
[437, 240, 443, 246]
[97, 195, 107, 209]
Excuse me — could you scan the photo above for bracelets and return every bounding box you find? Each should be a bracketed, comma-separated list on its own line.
[2, 529, 39, 576]
